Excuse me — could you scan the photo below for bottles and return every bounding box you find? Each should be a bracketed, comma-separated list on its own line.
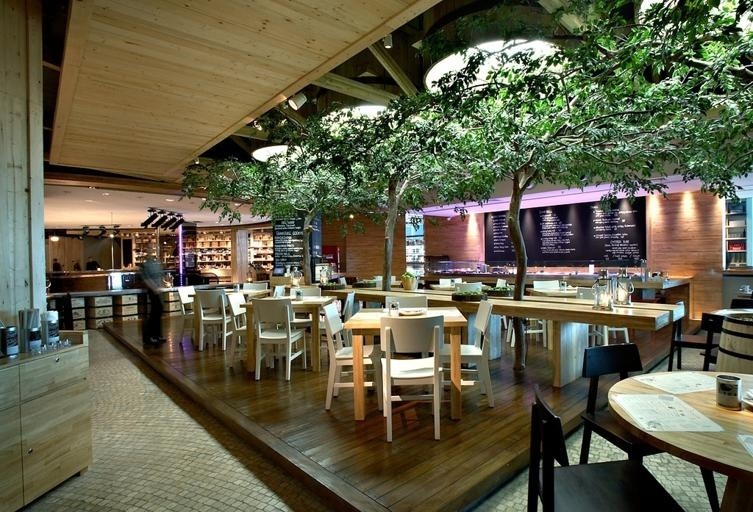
[290, 267, 302, 288]
[320, 266, 333, 284]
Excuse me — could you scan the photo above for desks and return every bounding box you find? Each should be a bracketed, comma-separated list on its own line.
[608, 371, 753, 511]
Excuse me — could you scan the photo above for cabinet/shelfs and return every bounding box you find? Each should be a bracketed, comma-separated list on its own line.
[46, 286, 166, 336]
[128, 229, 275, 271]
[0, 327, 95, 510]
[726, 197, 746, 270]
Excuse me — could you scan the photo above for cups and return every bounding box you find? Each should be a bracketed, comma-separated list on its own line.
[233, 283, 239, 292]
[715, 374, 743, 411]
[29, 338, 71, 356]
[296, 291, 303, 301]
[389, 302, 399, 317]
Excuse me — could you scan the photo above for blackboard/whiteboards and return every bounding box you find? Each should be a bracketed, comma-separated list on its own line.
[273, 218, 306, 276]
[484, 196, 646, 267]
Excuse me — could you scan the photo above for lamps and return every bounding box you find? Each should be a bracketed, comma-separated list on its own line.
[77, 224, 122, 240]
[138, 206, 186, 232]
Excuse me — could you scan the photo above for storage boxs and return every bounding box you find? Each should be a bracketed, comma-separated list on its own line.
[71, 295, 139, 332]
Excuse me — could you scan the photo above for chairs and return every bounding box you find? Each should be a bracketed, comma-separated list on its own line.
[579, 342, 723, 511]
[165, 276, 753, 443]
[526, 384, 684, 512]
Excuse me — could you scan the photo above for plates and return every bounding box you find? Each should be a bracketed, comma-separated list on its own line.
[429, 284, 454, 291]
[399, 309, 424, 315]
[740, 387, 753, 408]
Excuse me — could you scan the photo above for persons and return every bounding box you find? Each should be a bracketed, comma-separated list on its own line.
[53, 259, 62, 272]
[139, 242, 169, 350]
[73, 260, 81, 272]
[86, 256, 97, 270]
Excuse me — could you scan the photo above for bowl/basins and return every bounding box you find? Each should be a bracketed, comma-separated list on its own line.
[400, 276, 420, 291]
